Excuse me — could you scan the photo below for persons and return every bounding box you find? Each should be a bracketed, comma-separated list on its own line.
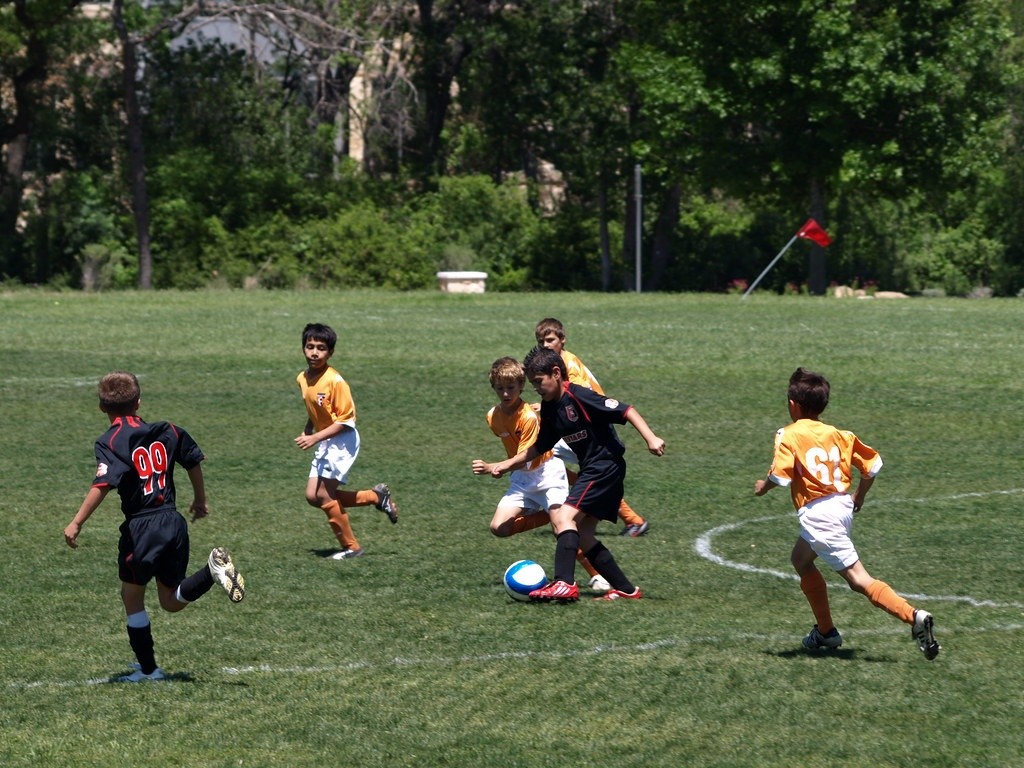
[472, 318, 666, 601]
[755, 367, 940, 660]
[64, 372, 245, 682]
[294, 322, 398, 560]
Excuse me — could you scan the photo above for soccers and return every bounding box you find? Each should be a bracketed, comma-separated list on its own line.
[503, 560, 547, 604]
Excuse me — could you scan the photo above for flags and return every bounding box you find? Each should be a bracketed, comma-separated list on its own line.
[796, 219, 832, 248]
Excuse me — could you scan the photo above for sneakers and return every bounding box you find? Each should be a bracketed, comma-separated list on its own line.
[117, 661, 165, 683]
[326, 547, 365, 560]
[372, 481, 400, 525]
[618, 519, 650, 537]
[587, 574, 611, 593]
[912, 608, 939, 661]
[529, 580, 580, 602]
[593, 586, 642, 601]
[207, 546, 245, 604]
[799, 624, 842, 650]
